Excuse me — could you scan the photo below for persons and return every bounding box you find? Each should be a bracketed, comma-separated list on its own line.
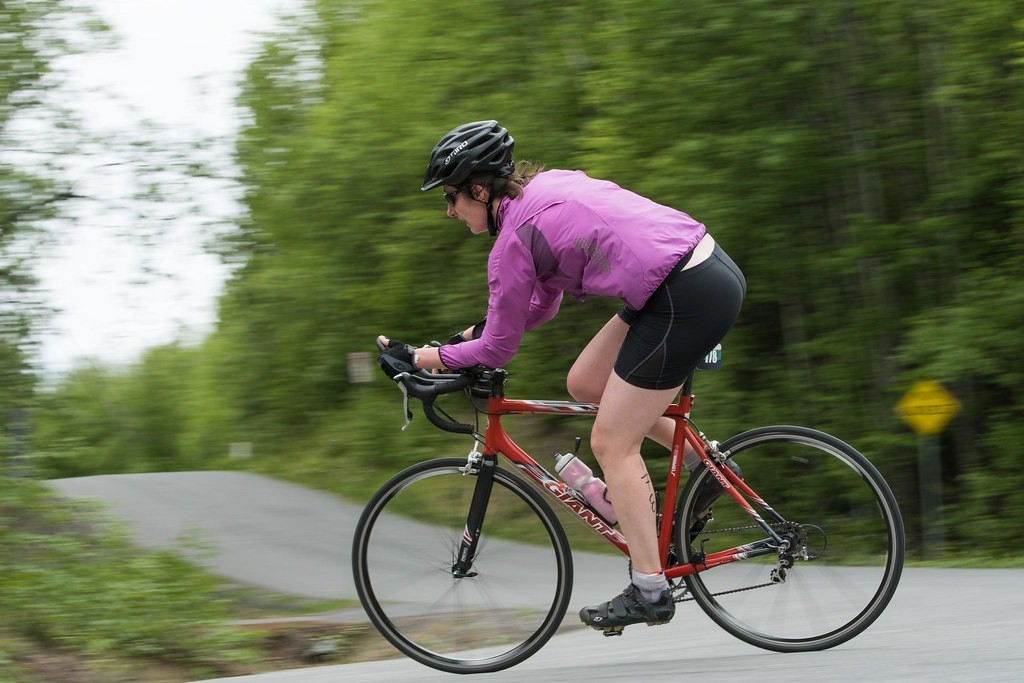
[378, 120, 747, 631]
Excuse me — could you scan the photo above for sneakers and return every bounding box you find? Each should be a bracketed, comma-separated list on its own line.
[579, 583, 675, 632]
[692, 457, 744, 520]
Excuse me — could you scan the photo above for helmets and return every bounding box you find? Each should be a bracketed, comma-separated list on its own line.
[421, 119, 515, 191]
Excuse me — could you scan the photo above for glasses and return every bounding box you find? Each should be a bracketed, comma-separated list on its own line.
[443, 183, 477, 207]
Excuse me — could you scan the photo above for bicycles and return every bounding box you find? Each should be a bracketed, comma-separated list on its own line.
[351, 335, 907, 675]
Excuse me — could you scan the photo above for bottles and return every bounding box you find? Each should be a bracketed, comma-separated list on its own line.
[554, 452, 623, 529]
